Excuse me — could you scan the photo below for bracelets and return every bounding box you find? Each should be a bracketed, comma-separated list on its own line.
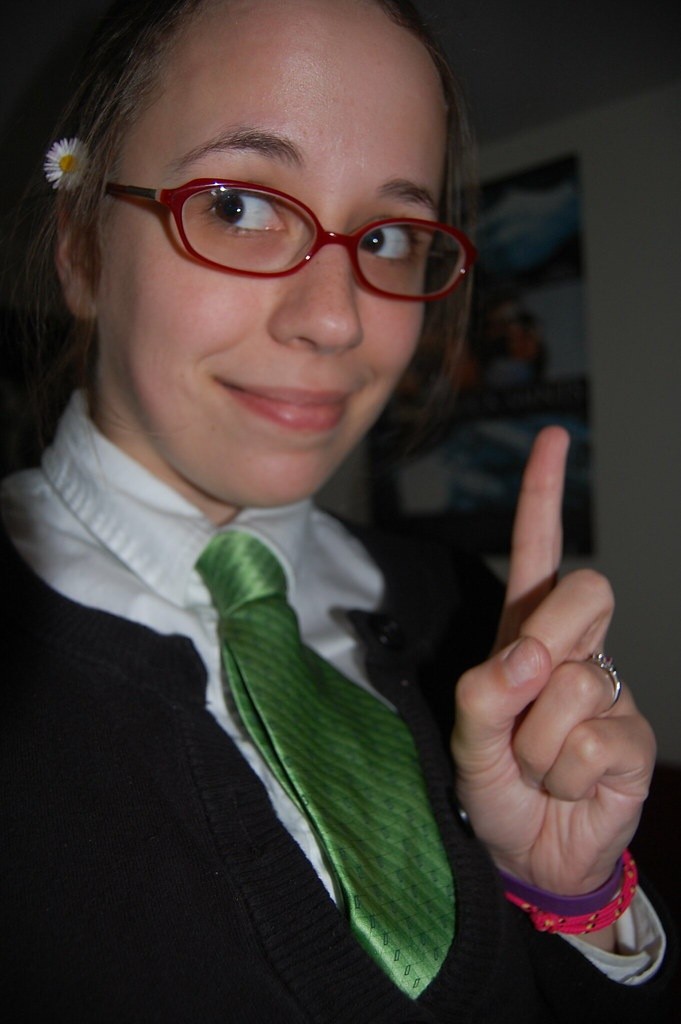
[501, 850, 636, 937]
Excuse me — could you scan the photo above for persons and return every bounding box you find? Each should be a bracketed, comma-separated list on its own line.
[1, 0, 681, 1024]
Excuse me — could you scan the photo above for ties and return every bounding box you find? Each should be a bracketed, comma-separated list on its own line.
[194, 530, 455, 1000]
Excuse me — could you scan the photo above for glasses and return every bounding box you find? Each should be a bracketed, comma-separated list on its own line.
[106, 178, 478, 302]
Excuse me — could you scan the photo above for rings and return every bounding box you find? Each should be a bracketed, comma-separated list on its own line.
[588, 656, 621, 707]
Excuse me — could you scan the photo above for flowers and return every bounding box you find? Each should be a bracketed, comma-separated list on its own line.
[43, 138, 90, 192]
